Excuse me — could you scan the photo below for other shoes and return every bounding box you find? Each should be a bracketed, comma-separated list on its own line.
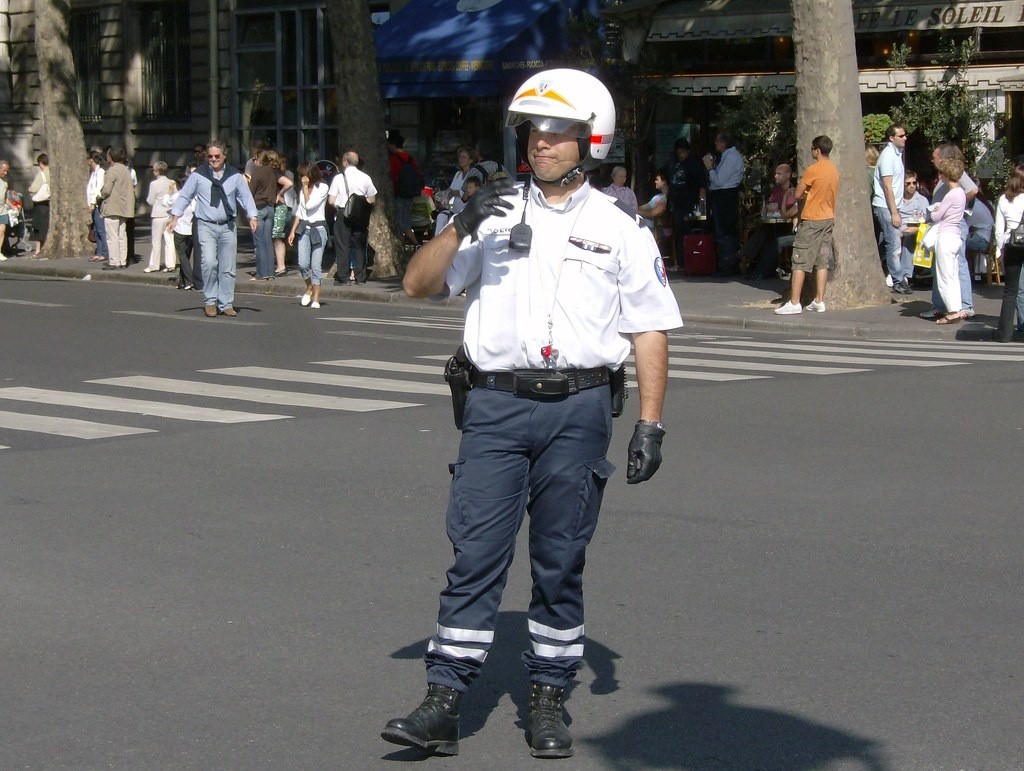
[301, 291, 320, 308]
[920, 309, 942, 318]
[205, 304, 237, 317]
[961, 309, 977, 320]
[88, 255, 107, 262]
[274, 268, 287, 277]
[102, 265, 128, 270]
[143, 267, 160, 273]
[162, 268, 176, 273]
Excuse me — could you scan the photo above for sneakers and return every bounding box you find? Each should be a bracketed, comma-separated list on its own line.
[892, 280, 913, 295]
[774, 300, 803, 314]
[804, 298, 826, 312]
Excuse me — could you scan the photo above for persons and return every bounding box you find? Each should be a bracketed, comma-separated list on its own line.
[774, 135, 840, 315]
[379, 67, 670, 757]
[584, 138, 697, 272]
[701, 132, 744, 283]
[744, 163, 799, 280]
[29, 153, 50, 260]
[166, 141, 259, 317]
[866, 123, 1024, 343]
[143, 161, 178, 272]
[412, 140, 531, 244]
[387, 134, 422, 253]
[0, 160, 19, 262]
[243, 139, 298, 282]
[171, 144, 204, 293]
[329, 150, 376, 285]
[86, 144, 138, 270]
[287, 161, 330, 308]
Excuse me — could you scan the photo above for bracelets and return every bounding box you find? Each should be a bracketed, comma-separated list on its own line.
[708, 168, 715, 172]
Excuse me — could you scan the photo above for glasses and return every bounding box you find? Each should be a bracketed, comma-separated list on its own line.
[810, 146, 820, 150]
[208, 154, 220, 159]
[194, 150, 203, 153]
[253, 159, 257, 162]
[892, 134, 906, 138]
[906, 181, 917, 185]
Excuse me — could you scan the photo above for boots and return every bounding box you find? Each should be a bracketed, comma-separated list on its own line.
[381, 682, 461, 755]
[525, 681, 574, 757]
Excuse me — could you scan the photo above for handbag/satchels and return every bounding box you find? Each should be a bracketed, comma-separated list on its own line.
[31, 172, 52, 202]
[86, 222, 97, 243]
[343, 193, 373, 232]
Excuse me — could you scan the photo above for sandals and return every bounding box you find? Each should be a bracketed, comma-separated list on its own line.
[936, 314, 961, 324]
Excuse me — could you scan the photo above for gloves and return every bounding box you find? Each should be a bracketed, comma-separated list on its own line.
[453, 173, 519, 240]
[626, 424, 667, 485]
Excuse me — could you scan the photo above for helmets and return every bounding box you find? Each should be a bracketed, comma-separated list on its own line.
[508, 69, 616, 160]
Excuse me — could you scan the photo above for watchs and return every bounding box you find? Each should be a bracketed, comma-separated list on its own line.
[636, 419, 664, 430]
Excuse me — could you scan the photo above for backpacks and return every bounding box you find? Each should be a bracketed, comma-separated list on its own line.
[392, 154, 422, 199]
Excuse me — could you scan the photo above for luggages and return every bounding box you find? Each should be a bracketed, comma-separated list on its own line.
[682, 228, 716, 275]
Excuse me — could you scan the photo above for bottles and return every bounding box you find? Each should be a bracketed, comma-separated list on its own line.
[760, 195, 767, 218]
[693, 203, 702, 216]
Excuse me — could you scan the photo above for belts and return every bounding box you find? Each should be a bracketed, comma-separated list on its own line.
[471, 366, 610, 392]
[201, 218, 234, 225]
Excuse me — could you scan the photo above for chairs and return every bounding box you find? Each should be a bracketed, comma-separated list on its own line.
[653, 212, 680, 269]
[962, 224, 1002, 286]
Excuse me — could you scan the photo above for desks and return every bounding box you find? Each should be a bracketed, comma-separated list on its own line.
[753, 216, 795, 279]
